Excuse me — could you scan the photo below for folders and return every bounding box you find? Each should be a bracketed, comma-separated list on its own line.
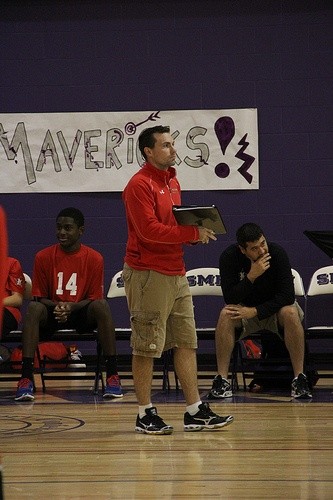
[171, 204, 228, 235]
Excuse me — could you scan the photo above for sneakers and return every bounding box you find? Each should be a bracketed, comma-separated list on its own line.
[14, 378, 34, 401]
[68, 345, 87, 368]
[206, 374, 233, 398]
[135, 407, 174, 435]
[102, 375, 124, 398]
[291, 373, 313, 398]
[184, 402, 234, 432]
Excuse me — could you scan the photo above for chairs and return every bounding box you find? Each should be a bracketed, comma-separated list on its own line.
[2, 265, 333, 394]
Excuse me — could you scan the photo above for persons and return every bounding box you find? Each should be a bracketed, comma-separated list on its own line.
[208, 222, 314, 399]
[122, 126, 234, 436]
[0, 208, 124, 401]
